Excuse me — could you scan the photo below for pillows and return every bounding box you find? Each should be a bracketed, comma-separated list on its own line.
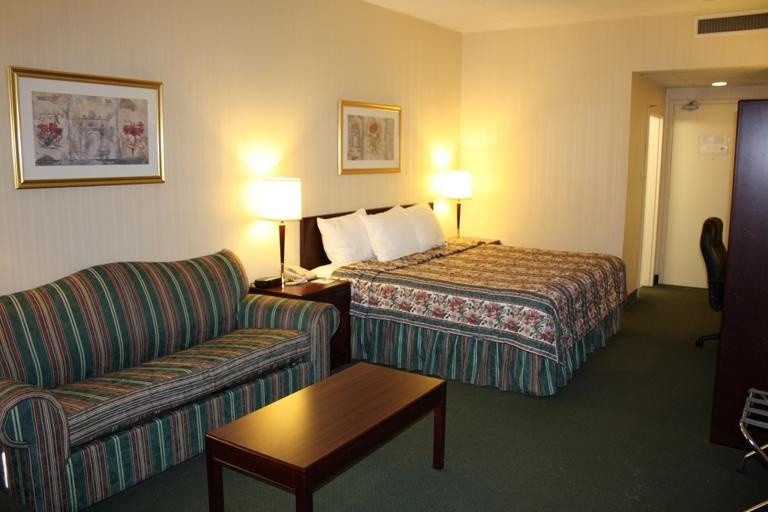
[316, 203, 446, 264]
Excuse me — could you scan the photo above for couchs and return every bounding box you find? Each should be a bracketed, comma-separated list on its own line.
[0, 248, 341, 512]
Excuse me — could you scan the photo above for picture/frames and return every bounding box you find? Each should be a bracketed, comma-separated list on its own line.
[336, 97, 403, 175]
[7, 64, 165, 189]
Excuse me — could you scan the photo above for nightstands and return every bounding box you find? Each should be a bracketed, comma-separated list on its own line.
[248, 276, 353, 371]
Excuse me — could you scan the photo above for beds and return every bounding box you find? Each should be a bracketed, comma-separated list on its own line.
[300, 202, 627, 399]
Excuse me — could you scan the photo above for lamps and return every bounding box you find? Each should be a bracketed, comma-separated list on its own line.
[256, 176, 301, 286]
[436, 170, 473, 241]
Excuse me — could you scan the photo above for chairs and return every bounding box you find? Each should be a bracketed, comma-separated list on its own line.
[694, 215, 730, 346]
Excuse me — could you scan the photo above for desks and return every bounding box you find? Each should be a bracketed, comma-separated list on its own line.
[202, 363, 448, 509]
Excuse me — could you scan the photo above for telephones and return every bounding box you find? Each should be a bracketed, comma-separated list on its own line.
[284, 266, 316, 280]
